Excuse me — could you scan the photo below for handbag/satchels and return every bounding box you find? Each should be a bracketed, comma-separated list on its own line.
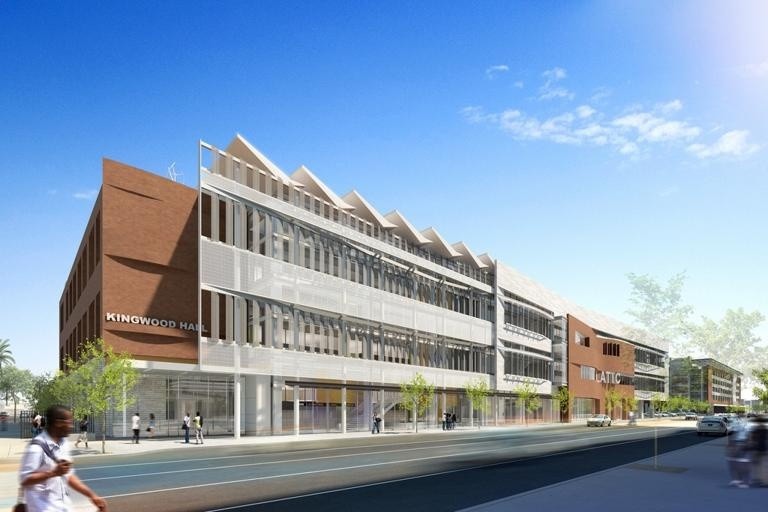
[182, 424, 187, 429]
[13, 503, 28, 512]
[146, 427, 150, 432]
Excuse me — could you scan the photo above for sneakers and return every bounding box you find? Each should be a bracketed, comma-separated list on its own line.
[728, 478, 768, 488]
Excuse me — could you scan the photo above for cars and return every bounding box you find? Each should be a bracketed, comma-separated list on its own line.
[629, 411, 744, 436]
[0, 411, 10, 417]
[587, 414, 611, 427]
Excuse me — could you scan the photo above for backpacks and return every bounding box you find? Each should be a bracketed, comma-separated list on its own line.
[41, 418, 45, 427]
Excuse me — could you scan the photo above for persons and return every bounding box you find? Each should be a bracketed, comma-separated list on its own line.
[193, 412, 204, 444]
[131, 413, 140, 444]
[184, 413, 190, 443]
[148, 413, 155, 439]
[442, 413, 456, 430]
[372, 412, 380, 434]
[19, 405, 107, 512]
[31, 411, 43, 438]
[75, 414, 89, 448]
